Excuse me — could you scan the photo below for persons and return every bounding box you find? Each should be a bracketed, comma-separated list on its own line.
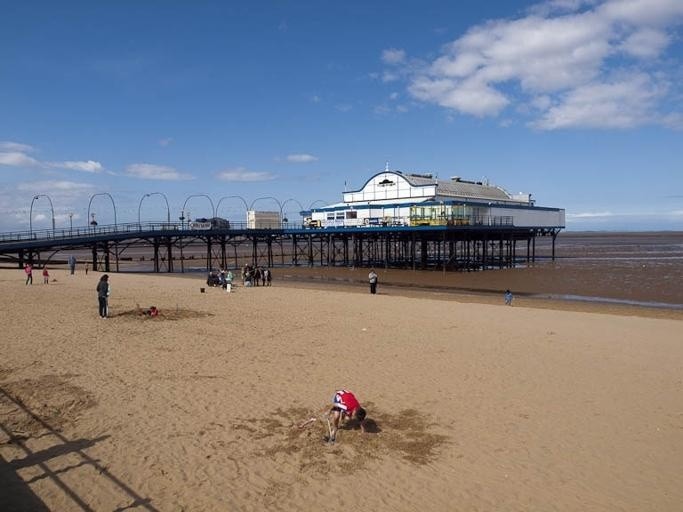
[95, 274, 110, 319]
[67, 254, 75, 276]
[328, 389, 367, 434]
[84, 262, 88, 275]
[205, 262, 271, 289]
[504, 289, 512, 305]
[41, 264, 48, 284]
[23, 262, 31, 286]
[367, 269, 377, 294]
